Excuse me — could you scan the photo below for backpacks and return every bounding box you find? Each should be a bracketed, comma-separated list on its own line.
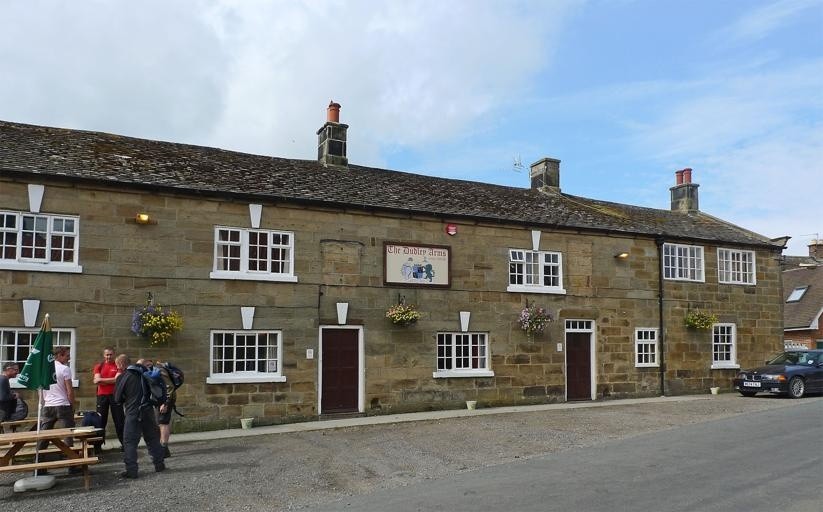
[162, 362, 184, 390]
[12, 397, 28, 420]
[81, 411, 106, 446]
[127, 364, 167, 407]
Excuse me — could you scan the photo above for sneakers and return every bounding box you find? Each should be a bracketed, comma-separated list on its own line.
[67, 466, 83, 474]
[155, 463, 166, 472]
[123, 470, 138, 478]
[33, 467, 48, 475]
[164, 448, 170, 458]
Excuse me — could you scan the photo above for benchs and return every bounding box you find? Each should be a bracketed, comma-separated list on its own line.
[0, 411, 104, 491]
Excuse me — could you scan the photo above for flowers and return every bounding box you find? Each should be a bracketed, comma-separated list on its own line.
[385, 302, 421, 328]
[517, 304, 554, 332]
[682, 309, 718, 329]
[131, 301, 184, 348]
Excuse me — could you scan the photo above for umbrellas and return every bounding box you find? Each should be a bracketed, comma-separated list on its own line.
[14, 314, 58, 480]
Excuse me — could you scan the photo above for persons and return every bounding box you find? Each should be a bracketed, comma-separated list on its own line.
[110, 353, 165, 477]
[91, 345, 124, 454]
[135, 356, 177, 458]
[33, 345, 84, 477]
[0, 360, 18, 432]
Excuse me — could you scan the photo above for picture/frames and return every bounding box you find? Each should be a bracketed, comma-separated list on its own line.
[382, 240, 452, 288]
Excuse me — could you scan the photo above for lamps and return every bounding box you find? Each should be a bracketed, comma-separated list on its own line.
[614, 252, 629, 258]
[126, 214, 157, 225]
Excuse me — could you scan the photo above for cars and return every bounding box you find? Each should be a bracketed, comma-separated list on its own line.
[733, 349, 823, 399]
